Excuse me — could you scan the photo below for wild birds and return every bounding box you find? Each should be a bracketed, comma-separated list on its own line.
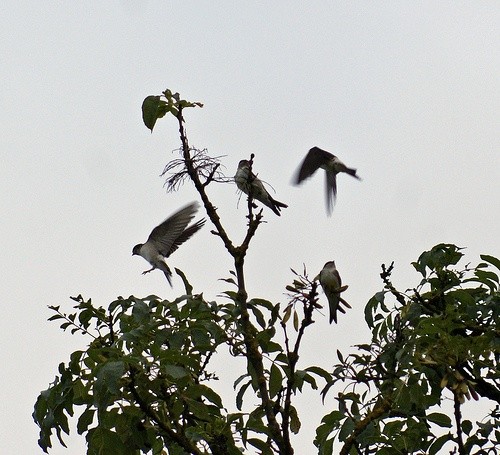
[293, 146, 359, 217]
[429, 369, 448, 405]
[131, 201, 206, 289]
[234, 160, 288, 217]
[320, 261, 342, 324]
